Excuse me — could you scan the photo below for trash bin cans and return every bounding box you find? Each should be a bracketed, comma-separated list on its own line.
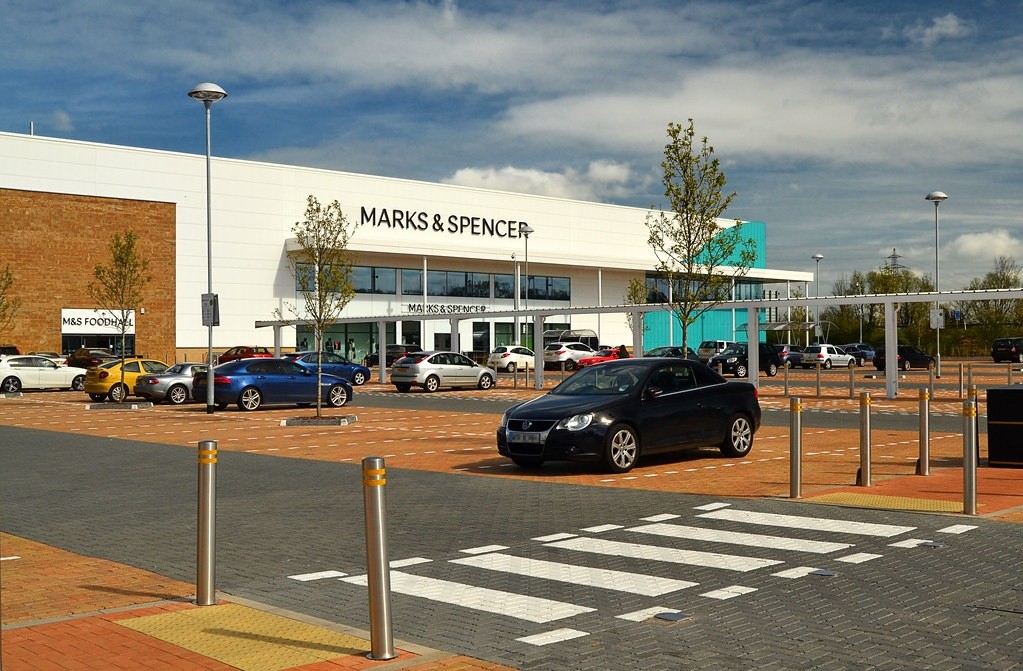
[986, 384, 1023, 469]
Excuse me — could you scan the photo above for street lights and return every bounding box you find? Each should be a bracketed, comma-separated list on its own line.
[511, 252, 517, 345]
[189, 83, 228, 415]
[811, 254, 824, 343]
[518, 226, 535, 347]
[925, 191, 948, 378]
[855, 282, 863, 343]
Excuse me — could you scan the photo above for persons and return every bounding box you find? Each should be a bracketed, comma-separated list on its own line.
[325, 338, 333, 362]
[347, 338, 352, 363]
[300, 338, 308, 351]
[618, 345, 629, 359]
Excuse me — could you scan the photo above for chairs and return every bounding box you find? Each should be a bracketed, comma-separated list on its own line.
[440, 357, 447, 364]
[654, 372, 678, 393]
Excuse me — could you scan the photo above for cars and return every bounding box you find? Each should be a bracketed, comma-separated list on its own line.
[390, 350, 497, 393]
[495, 356, 762, 473]
[487, 346, 545, 373]
[543, 341, 606, 371]
[773, 343, 874, 370]
[642, 347, 706, 364]
[697, 341, 736, 362]
[364, 344, 423, 368]
[576, 349, 635, 371]
[707, 342, 780, 377]
[0, 347, 371, 411]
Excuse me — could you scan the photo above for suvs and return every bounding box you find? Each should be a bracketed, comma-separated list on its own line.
[873, 345, 936, 371]
[991, 337, 1023, 363]
